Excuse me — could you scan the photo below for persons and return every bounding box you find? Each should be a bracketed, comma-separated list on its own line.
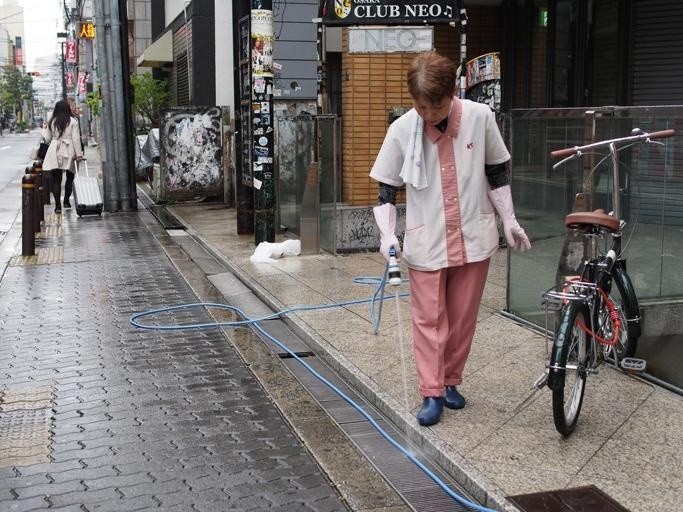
[369, 51, 532, 425]
[42, 100, 83, 213]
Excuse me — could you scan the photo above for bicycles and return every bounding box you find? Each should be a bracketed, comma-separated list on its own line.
[514, 128, 674, 435]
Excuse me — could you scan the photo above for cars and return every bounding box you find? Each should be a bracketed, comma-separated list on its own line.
[33, 116, 43, 128]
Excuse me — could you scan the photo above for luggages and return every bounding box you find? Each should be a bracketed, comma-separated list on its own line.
[72, 158, 104, 217]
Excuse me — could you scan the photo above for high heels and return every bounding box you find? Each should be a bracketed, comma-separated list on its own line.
[55, 203, 71, 213]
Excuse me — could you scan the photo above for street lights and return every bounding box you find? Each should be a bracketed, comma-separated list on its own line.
[57, 32, 67, 100]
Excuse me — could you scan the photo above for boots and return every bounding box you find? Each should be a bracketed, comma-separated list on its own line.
[417, 385, 465, 425]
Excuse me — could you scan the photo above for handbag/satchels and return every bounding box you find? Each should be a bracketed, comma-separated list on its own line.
[37, 138, 49, 160]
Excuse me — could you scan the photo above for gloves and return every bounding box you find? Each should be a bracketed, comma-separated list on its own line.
[372, 203, 401, 263]
[487, 185, 532, 252]
